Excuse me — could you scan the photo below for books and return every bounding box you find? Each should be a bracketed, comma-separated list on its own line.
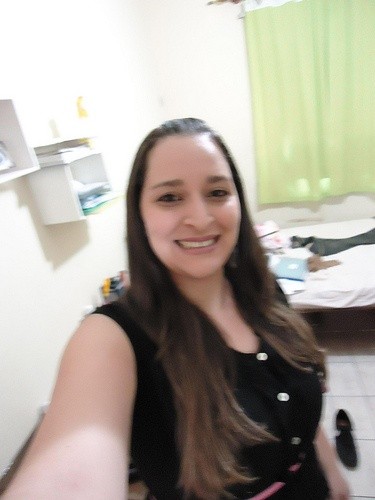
[75, 182, 117, 214]
[33, 135, 91, 155]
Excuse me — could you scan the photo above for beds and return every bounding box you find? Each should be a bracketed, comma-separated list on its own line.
[254, 219, 375, 326]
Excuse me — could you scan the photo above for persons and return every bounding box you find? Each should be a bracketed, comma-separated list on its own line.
[0, 117, 354, 500]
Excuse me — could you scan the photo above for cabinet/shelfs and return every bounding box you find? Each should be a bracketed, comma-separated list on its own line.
[0, 99, 120, 226]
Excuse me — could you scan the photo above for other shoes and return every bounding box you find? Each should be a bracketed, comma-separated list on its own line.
[336, 409, 357, 467]
